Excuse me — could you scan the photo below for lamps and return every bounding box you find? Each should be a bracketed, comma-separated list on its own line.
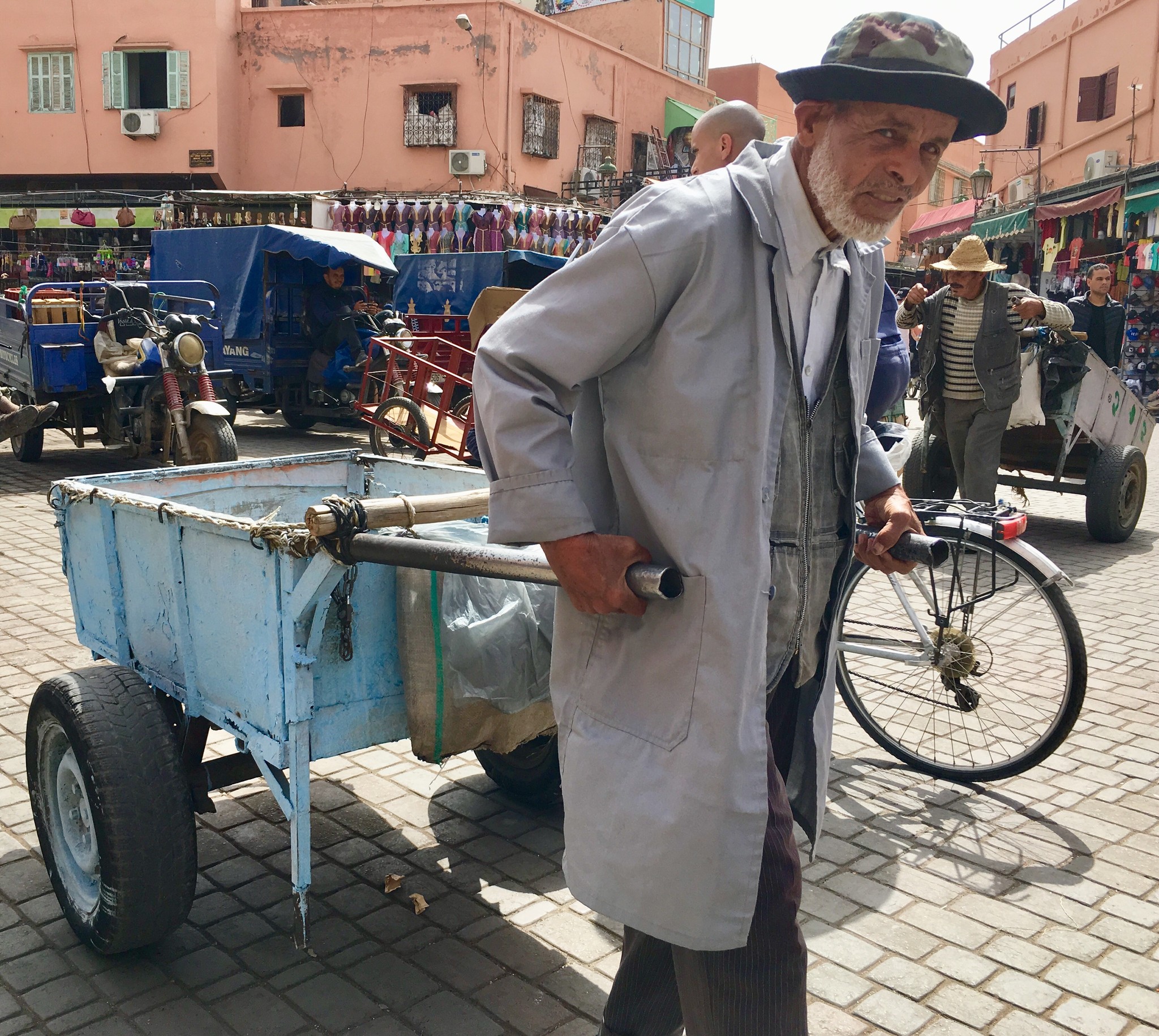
[572, 167, 601, 200]
[969, 147, 1041, 199]
[573, 144, 618, 202]
[456, 14, 479, 66]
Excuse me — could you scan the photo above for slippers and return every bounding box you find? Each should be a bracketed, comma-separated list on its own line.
[154, 208, 308, 229]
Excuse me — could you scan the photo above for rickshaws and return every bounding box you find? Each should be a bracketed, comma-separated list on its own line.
[902, 296, 1156, 543]
[26, 447, 950, 959]
[350, 335, 477, 461]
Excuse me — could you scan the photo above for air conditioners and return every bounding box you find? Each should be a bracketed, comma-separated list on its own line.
[449, 149, 485, 175]
[1083, 150, 1117, 181]
[1008, 175, 1034, 208]
[121, 110, 160, 136]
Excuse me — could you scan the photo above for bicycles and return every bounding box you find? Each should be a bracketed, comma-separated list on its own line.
[835, 421, 1088, 782]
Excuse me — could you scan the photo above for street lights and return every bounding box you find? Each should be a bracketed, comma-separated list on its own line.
[969, 147, 1040, 295]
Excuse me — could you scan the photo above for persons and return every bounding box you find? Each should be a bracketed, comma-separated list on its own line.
[1064, 261, 1126, 374]
[0, 393, 59, 444]
[502, 199, 601, 259]
[865, 278, 923, 433]
[688, 100, 767, 178]
[894, 236, 1074, 552]
[1081, 231, 1116, 263]
[310, 265, 429, 368]
[330, 198, 505, 253]
[471, 13, 1005, 1036]
[984, 240, 1038, 291]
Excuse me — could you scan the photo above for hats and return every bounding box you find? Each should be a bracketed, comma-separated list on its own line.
[775, 12, 1007, 144]
[1124, 276, 1159, 390]
[929, 235, 1008, 271]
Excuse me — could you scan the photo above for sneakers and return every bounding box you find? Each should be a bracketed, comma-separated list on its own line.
[355, 350, 373, 367]
[11, 401, 60, 437]
[0, 404, 39, 443]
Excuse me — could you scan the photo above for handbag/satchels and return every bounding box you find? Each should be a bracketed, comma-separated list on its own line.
[71, 199, 96, 227]
[116, 198, 134, 228]
[9, 200, 36, 230]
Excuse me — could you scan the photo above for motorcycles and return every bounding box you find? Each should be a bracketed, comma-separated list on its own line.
[1, 223, 568, 468]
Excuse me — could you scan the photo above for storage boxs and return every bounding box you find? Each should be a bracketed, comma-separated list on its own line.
[4, 288, 79, 324]
